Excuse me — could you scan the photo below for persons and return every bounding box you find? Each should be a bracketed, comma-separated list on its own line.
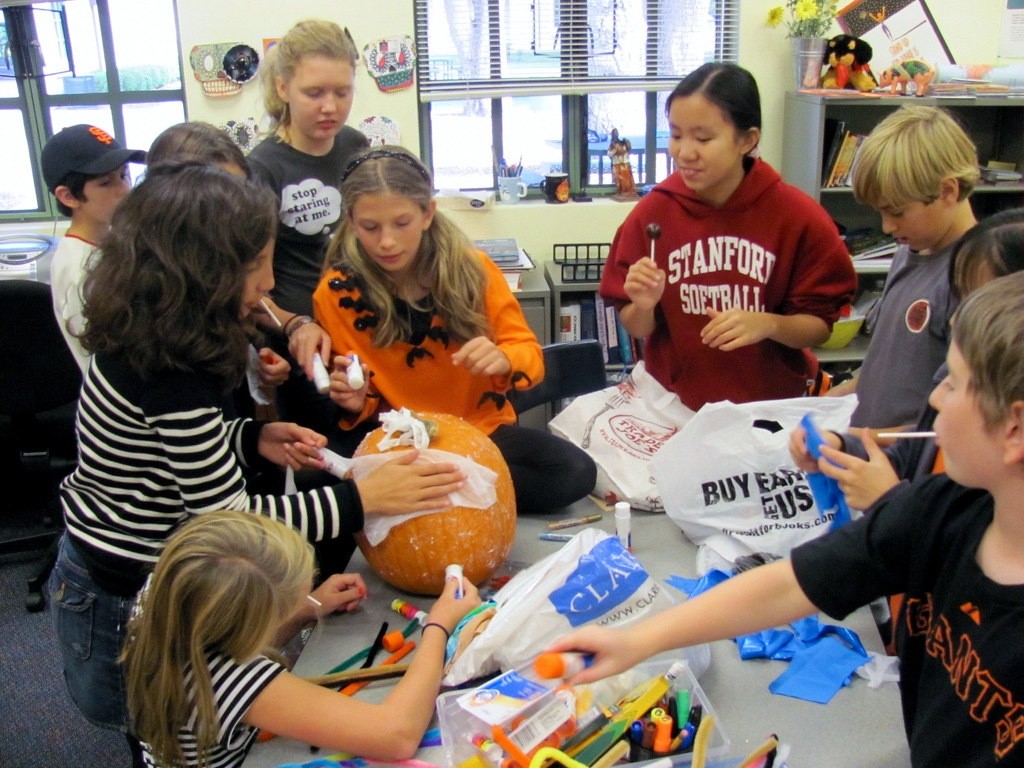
[608, 128, 637, 198]
[48, 156, 331, 768]
[544, 269, 1024, 768]
[146, 20, 375, 493]
[599, 61, 858, 413]
[312, 144, 597, 518]
[820, 105, 979, 429]
[49, 124, 146, 378]
[117, 511, 482, 768]
[789, 208, 1024, 654]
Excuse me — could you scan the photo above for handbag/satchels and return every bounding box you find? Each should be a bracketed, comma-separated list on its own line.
[645, 393, 860, 577]
[440, 528, 711, 687]
[548, 360, 697, 512]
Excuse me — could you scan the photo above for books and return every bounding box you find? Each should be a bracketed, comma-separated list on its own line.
[931, 76, 1009, 100]
[979, 160, 1023, 187]
[559, 293, 646, 365]
[844, 226, 899, 270]
[821, 119, 869, 189]
[471, 238, 536, 292]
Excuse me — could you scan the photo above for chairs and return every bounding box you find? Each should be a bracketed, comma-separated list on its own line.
[0, 278, 83, 614]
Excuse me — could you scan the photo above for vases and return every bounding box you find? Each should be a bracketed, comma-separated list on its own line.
[794, 38, 828, 90]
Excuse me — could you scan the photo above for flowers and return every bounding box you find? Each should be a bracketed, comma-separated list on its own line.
[766, 0, 841, 41]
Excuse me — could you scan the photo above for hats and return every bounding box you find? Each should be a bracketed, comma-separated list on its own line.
[41, 124, 146, 192]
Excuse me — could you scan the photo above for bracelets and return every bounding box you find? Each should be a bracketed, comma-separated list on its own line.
[422, 623, 449, 641]
[283, 314, 304, 335]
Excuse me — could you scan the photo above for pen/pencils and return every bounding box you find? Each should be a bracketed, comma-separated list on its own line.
[547, 513, 604, 529]
[539, 532, 577, 543]
[629, 689, 708, 763]
[498, 156, 522, 177]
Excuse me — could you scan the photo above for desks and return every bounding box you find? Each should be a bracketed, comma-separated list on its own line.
[237, 490, 911, 767]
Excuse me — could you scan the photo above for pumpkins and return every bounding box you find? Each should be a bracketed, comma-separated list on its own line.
[347, 411, 516, 596]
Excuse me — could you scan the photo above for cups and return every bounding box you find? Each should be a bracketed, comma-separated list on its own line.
[497, 175, 527, 203]
[539, 172, 569, 204]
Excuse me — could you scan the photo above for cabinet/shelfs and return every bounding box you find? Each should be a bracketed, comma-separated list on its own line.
[783, 88, 1024, 389]
[544, 260, 645, 416]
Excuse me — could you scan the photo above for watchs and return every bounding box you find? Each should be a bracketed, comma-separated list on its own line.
[287, 315, 319, 339]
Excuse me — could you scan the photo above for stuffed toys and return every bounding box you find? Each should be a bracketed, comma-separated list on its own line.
[821, 34, 877, 93]
